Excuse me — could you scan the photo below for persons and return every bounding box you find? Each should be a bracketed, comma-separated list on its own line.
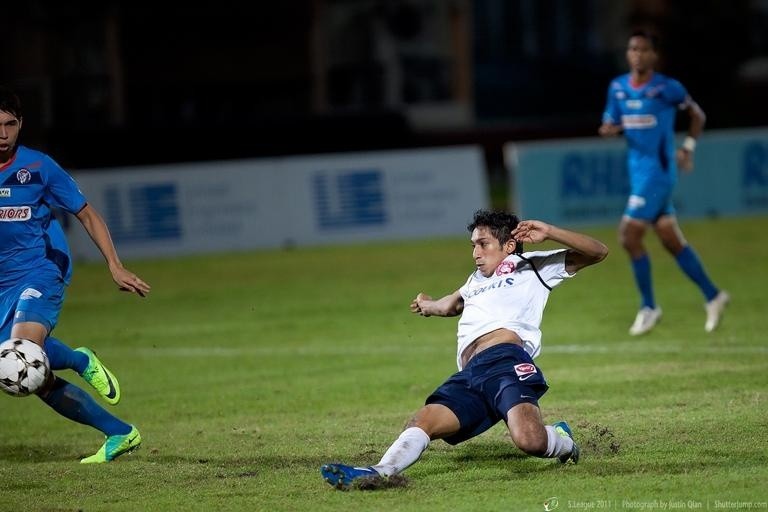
[598, 36, 731, 336]
[0, 95, 151, 462]
[319, 208, 608, 491]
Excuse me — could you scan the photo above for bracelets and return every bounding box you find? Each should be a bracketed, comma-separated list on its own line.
[683, 137, 696, 151]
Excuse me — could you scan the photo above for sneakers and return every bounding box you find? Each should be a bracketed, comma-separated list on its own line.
[80, 424, 142, 464]
[703, 289, 730, 332]
[318, 463, 380, 491]
[74, 347, 121, 406]
[627, 304, 663, 337]
[551, 420, 579, 463]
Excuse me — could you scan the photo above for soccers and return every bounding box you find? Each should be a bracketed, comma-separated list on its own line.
[0, 337, 50, 396]
